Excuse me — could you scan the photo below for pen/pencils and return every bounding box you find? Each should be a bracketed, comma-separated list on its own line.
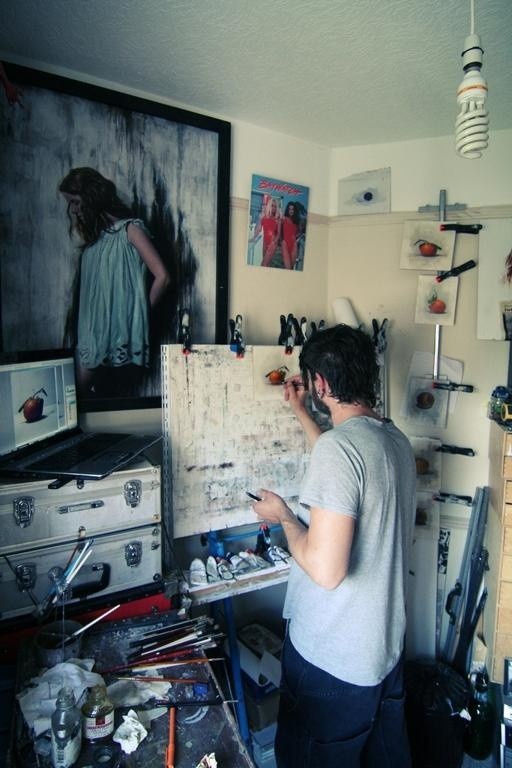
[156, 700, 223, 706]
[165, 707, 176, 768]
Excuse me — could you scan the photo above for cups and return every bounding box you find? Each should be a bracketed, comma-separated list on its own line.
[486, 387, 509, 423]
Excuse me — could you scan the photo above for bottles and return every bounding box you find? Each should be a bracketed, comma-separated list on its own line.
[49, 685, 84, 768]
[87, 687, 116, 743]
[466, 671, 495, 761]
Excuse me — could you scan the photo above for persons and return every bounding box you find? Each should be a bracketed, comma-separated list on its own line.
[248, 197, 301, 270]
[59, 167, 170, 395]
[253, 324, 418, 767]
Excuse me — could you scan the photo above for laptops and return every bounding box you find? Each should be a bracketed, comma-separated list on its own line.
[0, 348, 162, 480]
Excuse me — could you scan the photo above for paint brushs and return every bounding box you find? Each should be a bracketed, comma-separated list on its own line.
[40, 539, 94, 622]
[56, 603, 120, 649]
[95, 614, 227, 683]
[247, 492, 261, 501]
[281, 382, 304, 386]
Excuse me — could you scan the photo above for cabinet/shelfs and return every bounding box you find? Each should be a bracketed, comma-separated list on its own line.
[485, 420, 512, 688]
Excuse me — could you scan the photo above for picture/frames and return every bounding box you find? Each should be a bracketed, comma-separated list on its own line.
[0, 60, 232, 415]
